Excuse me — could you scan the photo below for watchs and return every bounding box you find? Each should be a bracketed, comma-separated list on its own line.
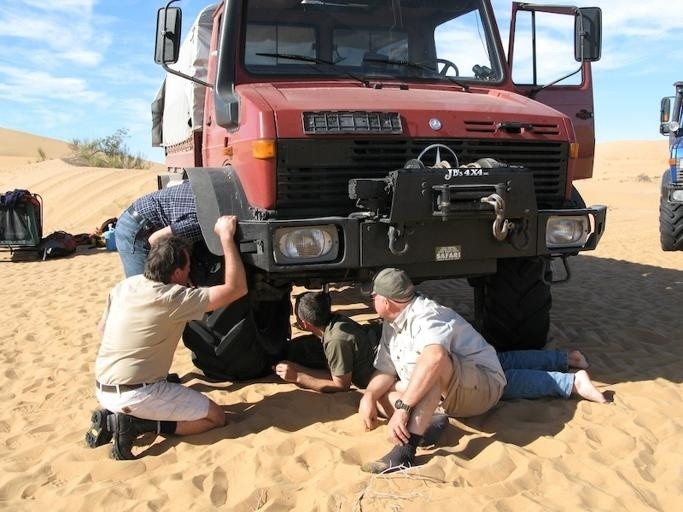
[394, 399, 413, 415]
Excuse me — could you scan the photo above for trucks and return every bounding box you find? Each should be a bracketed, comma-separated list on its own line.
[660, 82, 683, 249]
[151, 1, 602, 381]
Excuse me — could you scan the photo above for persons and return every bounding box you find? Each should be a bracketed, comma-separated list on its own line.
[84, 216, 248, 460]
[358, 268, 507, 474]
[115, 180, 205, 384]
[275, 291, 610, 405]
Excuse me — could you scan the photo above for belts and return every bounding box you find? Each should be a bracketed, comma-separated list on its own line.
[95, 380, 148, 393]
[128, 206, 152, 231]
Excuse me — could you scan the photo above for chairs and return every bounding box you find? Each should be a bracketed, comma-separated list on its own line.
[0, 192, 43, 262]
[361, 51, 389, 67]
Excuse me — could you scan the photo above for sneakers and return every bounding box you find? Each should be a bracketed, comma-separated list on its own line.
[85, 408, 113, 448]
[106, 412, 137, 460]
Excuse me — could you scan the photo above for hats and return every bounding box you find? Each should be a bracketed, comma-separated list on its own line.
[361, 267, 414, 299]
